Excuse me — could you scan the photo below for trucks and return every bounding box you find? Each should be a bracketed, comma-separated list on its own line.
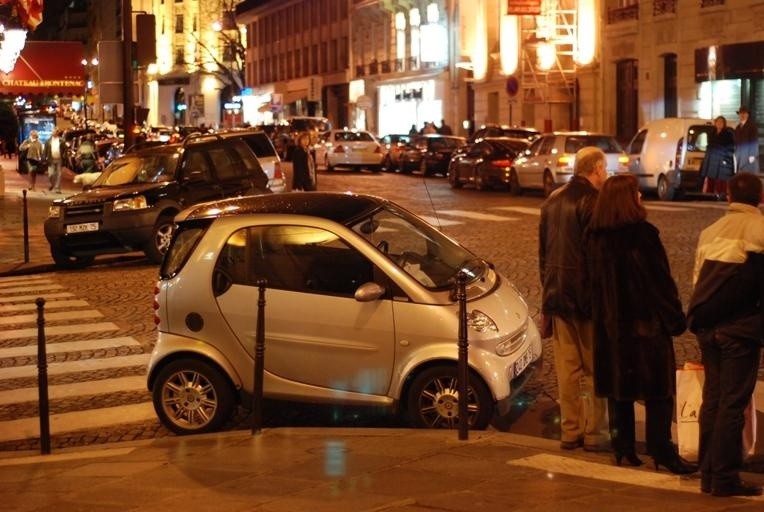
[19, 112, 56, 149]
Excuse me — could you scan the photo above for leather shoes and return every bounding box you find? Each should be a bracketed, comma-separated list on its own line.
[560, 439, 610, 451]
[700, 473, 761, 497]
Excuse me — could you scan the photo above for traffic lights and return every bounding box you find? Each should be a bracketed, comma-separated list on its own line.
[175, 87, 185, 114]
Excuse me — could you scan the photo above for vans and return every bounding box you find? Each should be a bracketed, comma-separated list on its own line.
[624, 116, 737, 203]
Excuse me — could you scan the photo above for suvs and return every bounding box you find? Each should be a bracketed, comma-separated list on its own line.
[257, 115, 331, 150]
[450, 124, 539, 157]
[183, 130, 287, 194]
[398, 135, 465, 176]
[42, 141, 273, 268]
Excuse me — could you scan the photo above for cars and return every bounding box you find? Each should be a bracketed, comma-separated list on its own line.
[57, 126, 210, 175]
[378, 134, 417, 171]
[509, 131, 630, 197]
[46, 99, 100, 129]
[312, 129, 382, 173]
[143, 190, 541, 428]
[447, 137, 531, 191]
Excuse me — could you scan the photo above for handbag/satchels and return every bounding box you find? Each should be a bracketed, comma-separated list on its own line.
[675, 362, 756, 462]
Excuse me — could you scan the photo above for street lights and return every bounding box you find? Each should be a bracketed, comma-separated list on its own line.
[214, 22, 236, 129]
[707, 47, 720, 118]
[80, 49, 101, 120]
[13, 96, 39, 117]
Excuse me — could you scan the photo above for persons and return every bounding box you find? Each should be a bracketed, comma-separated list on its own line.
[700, 115, 735, 204]
[733, 107, 761, 175]
[410, 119, 451, 135]
[539, 146, 617, 451]
[686, 173, 764, 496]
[290, 130, 317, 193]
[42, 126, 69, 195]
[18, 130, 42, 192]
[582, 175, 700, 476]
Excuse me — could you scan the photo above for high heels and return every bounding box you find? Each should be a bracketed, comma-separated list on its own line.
[654, 448, 698, 472]
[613, 446, 643, 466]
